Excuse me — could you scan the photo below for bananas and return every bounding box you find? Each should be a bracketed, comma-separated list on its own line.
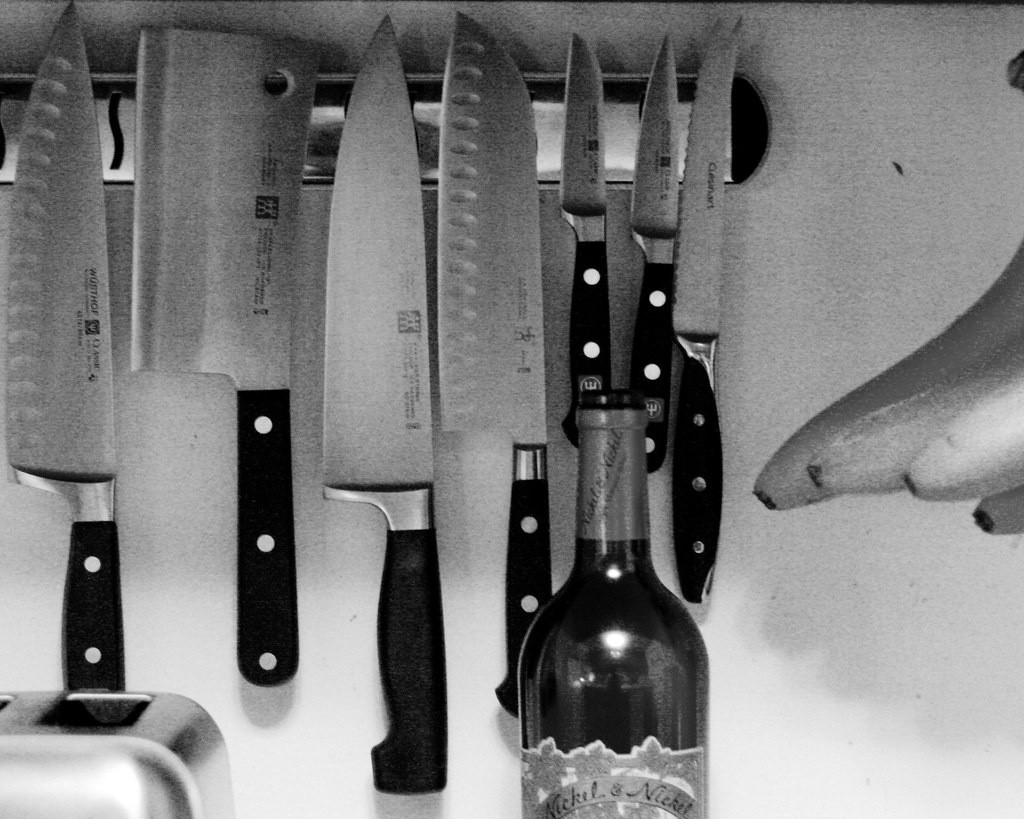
[750, 242, 1024, 534]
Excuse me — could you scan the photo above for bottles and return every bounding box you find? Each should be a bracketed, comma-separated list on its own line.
[517, 390, 710, 819]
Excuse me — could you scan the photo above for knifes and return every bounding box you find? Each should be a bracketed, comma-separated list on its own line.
[322, 13, 447, 797]
[433, 9, 553, 719]
[131, 28, 325, 688]
[0, 1, 125, 690]
[674, 11, 746, 604]
[559, 32, 610, 448]
[629, 31, 681, 472]
